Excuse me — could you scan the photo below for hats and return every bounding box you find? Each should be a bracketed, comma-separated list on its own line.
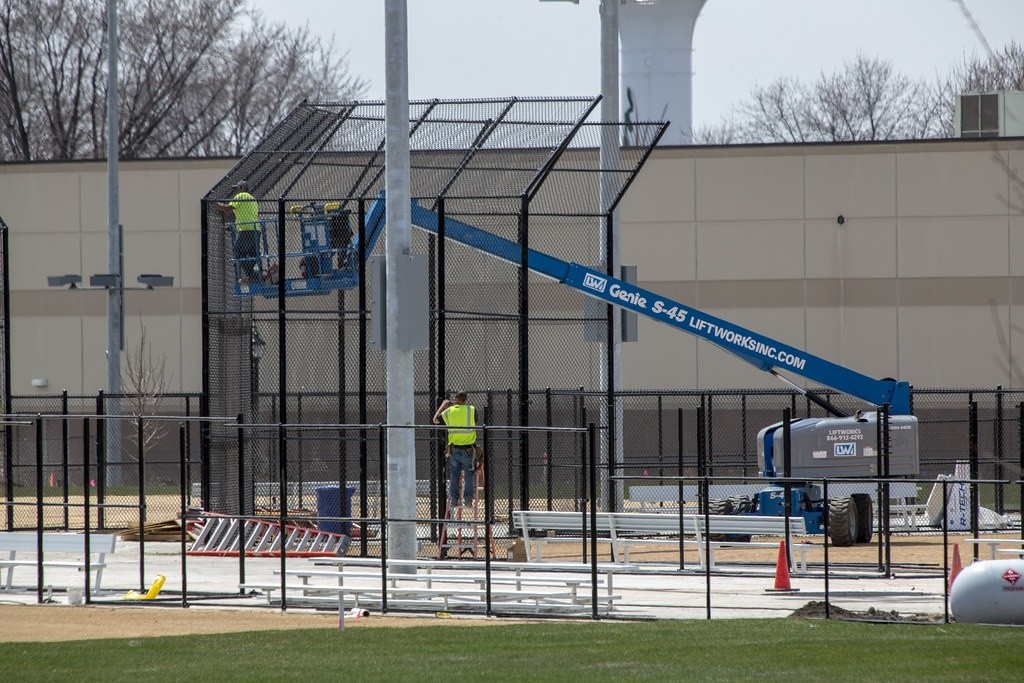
[232, 181, 250, 189]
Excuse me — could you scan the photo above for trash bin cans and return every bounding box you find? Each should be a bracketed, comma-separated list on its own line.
[315, 485, 357, 535]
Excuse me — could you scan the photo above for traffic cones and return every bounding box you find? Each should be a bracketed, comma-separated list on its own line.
[763, 538, 802, 593]
[942, 543, 964, 599]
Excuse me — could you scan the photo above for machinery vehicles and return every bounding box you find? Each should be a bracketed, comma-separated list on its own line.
[227, 192, 920, 543]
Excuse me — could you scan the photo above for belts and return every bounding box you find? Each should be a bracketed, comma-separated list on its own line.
[453, 444, 476, 449]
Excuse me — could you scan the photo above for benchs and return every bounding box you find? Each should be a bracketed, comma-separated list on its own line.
[965, 539, 1024, 560]
[238, 567, 625, 612]
[513, 510, 823, 568]
[1, 531, 119, 597]
[812, 482, 942, 531]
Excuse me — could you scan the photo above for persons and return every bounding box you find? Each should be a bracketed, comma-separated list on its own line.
[215, 180, 260, 284]
[432, 390, 478, 507]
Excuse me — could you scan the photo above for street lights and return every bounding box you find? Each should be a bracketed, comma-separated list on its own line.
[47, 276, 173, 493]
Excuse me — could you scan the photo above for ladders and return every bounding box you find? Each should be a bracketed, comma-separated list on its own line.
[186, 511, 351, 557]
[438, 464, 496, 561]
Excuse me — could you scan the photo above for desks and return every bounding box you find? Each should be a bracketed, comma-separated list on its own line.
[629, 484, 792, 514]
